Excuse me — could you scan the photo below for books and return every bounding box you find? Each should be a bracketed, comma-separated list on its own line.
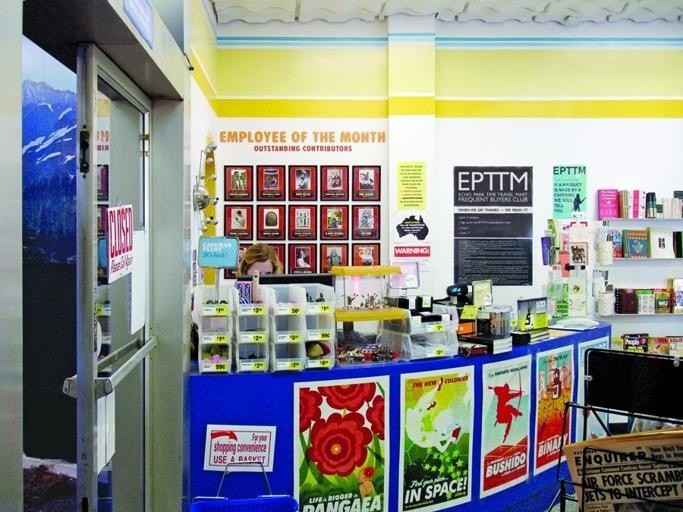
[458, 296, 550, 356]
[597, 189, 683, 362]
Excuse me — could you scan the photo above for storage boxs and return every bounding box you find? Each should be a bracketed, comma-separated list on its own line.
[331, 309, 407, 367]
[330, 264, 407, 312]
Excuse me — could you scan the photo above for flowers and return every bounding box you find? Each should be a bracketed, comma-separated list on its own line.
[300, 382, 384, 490]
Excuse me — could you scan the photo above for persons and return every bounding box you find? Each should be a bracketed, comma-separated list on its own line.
[231, 171, 244, 191]
[238, 244, 280, 276]
[235, 211, 246, 228]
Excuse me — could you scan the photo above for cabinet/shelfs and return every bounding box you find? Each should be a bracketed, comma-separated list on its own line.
[194, 283, 336, 372]
[595, 217, 683, 316]
[376, 298, 460, 361]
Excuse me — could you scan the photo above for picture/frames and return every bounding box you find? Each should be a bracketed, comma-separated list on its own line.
[223, 164, 381, 279]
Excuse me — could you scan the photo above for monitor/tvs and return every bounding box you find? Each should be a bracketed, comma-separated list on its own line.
[389, 259, 421, 296]
[472, 279, 494, 310]
[233, 273, 335, 290]
